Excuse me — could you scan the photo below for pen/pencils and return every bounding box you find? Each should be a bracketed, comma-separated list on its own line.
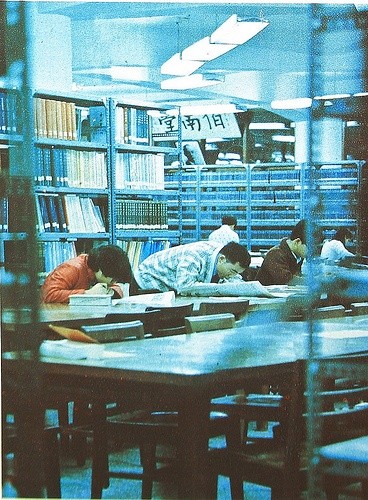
[102, 285, 106, 289]
[224, 278, 229, 283]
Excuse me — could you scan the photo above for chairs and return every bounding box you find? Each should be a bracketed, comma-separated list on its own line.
[82, 299, 368, 500]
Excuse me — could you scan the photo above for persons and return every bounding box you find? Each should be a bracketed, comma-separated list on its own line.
[210, 298, 211, 299]
[208, 216, 239, 242]
[133, 240, 251, 294]
[40, 244, 132, 303]
[320, 229, 358, 260]
[255, 218, 323, 286]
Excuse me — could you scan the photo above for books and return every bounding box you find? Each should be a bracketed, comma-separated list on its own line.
[38, 323, 106, 360]
[112, 290, 176, 307]
[0, 91, 358, 276]
[68, 293, 113, 307]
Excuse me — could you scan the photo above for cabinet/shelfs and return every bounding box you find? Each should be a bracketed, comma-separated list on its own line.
[165, 161, 366, 257]
[0, 86, 182, 289]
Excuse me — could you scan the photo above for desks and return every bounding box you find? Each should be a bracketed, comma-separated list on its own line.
[1, 286, 327, 464]
[1, 315, 368, 500]
